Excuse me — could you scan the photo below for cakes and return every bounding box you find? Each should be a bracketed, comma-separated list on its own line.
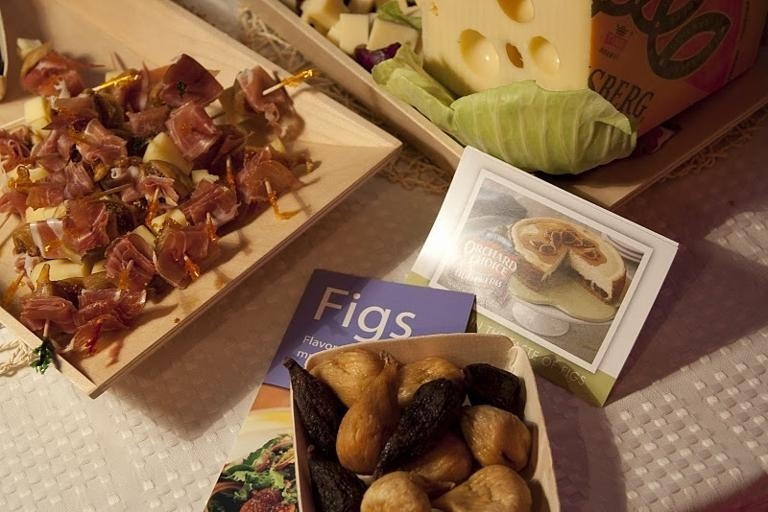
[505, 216, 626, 305]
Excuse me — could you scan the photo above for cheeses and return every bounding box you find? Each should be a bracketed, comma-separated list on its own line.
[300, 2, 768, 139]
